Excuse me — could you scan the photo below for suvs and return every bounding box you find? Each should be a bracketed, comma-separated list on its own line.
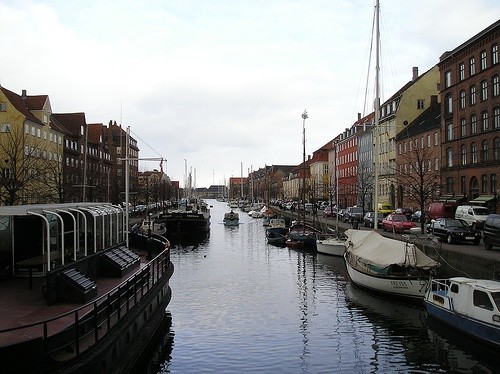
[341, 206, 365, 224]
[482, 214, 500, 250]
[323, 208, 337, 218]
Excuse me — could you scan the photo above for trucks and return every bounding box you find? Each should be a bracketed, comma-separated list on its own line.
[454, 205, 492, 233]
[377, 203, 396, 216]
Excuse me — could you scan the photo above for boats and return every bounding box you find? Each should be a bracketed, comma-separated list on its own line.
[315, 224, 345, 256]
[133, 219, 167, 236]
[423, 275, 500, 345]
[163, 195, 211, 234]
[183, 195, 210, 219]
[222, 212, 239, 226]
[229, 200, 287, 243]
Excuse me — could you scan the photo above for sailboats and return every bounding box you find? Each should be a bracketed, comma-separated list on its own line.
[343, 0, 440, 299]
[285, 107, 335, 250]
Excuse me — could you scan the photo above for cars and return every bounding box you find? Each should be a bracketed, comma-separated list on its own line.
[126, 198, 185, 214]
[392, 208, 414, 220]
[338, 211, 344, 221]
[272, 199, 336, 213]
[425, 217, 482, 246]
[411, 211, 428, 223]
[363, 212, 384, 228]
[382, 213, 416, 234]
